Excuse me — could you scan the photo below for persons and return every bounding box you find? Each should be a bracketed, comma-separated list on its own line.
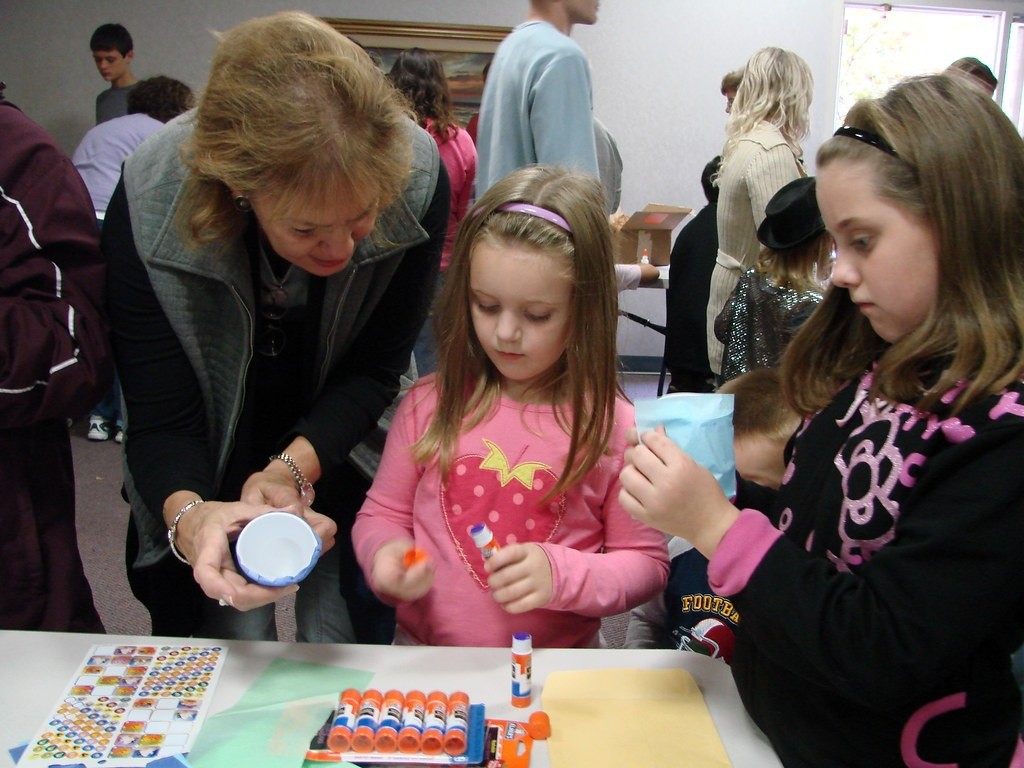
[351, 168, 670, 648]
[72, 22, 195, 441]
[714, 177, 831, 388]
[670, 46, 812, 395]
[619, 74, 1024, 768]
[0, 79, 108, 632]
[945, 57, 998, 98]
[389, 0, 660, 378]
[104, 11, 452, 645]
[626, 370, 801, 664]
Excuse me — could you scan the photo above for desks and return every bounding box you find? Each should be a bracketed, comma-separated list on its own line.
[0, 631, 785, 768]
[616, 268, 669, 397]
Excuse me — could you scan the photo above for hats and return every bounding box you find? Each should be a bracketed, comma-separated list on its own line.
[757, 173, 826, 253]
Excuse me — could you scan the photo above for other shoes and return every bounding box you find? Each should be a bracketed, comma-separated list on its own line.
[114, 431, 126, 442]
[87, 418, 111, 440]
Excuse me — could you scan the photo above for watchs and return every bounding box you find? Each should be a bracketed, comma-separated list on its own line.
[270, 453, 315, 507]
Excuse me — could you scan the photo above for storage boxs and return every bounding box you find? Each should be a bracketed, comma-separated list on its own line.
[620, 205, 695, 265]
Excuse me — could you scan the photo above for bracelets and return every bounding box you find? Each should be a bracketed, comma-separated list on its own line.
[167, 501, 203, 565]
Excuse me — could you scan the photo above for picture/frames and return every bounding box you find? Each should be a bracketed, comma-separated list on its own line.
[323, 17, 519, 122]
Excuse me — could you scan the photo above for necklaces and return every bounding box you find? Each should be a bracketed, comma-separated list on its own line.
[262, 244, 294, 319]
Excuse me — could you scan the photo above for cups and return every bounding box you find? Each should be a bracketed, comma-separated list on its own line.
[236, 511, 321, 588]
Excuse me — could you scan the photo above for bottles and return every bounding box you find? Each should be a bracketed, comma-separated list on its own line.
[641, 249, 649, 265]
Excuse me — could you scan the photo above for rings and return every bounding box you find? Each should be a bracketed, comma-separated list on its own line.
[219, 599, 228, 606]
